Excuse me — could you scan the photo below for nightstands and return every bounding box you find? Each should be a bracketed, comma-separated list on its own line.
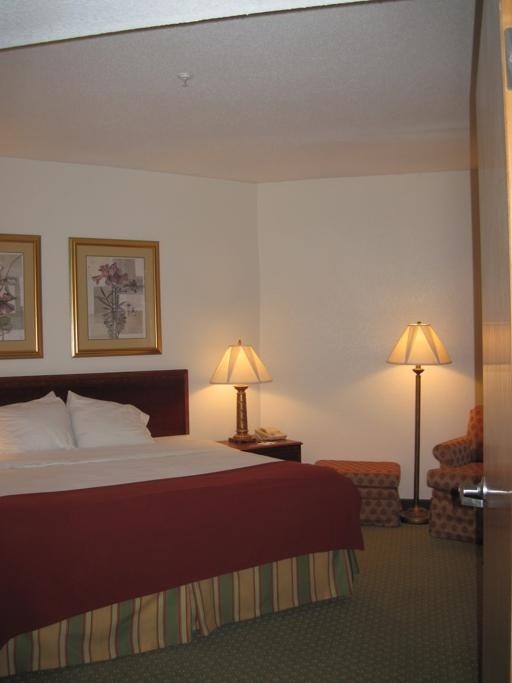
[215, 438, 303, 463]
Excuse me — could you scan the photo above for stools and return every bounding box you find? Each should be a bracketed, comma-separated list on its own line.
[314, 459, 404, 530]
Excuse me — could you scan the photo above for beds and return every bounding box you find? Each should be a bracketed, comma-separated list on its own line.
[0, 369, 366, 678]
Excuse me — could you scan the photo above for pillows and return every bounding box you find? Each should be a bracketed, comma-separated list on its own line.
[66, 390, 153, 438]
[0, 390, 77, 456]
[68, 397, 156, 449]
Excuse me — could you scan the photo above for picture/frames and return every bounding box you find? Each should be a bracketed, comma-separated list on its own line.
[68, 236, 163, 356]
[0, 233, 44, 360]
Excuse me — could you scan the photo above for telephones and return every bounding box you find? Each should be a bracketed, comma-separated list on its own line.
[254, 426, 287, 441]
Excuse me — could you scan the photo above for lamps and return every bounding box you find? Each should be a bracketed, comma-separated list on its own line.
[209, 339, 273, 444]
[386, 320, 453, 525]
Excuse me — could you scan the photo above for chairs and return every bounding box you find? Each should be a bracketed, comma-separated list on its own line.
[426, 405, 484, 544]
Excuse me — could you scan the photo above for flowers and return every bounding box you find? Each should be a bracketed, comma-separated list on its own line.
[92, 262, 144, 338]
